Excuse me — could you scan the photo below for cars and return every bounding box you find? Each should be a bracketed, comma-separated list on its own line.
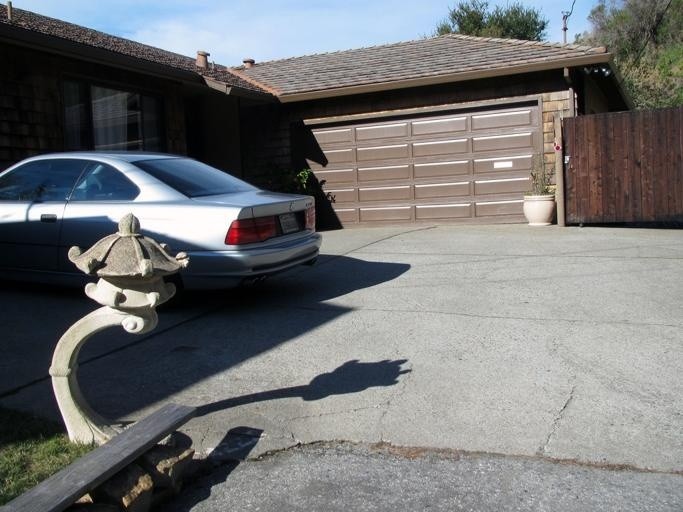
[0, 145, 322, 296]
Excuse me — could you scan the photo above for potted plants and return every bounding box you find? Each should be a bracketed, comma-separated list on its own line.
[523, 163, 557, 226]
